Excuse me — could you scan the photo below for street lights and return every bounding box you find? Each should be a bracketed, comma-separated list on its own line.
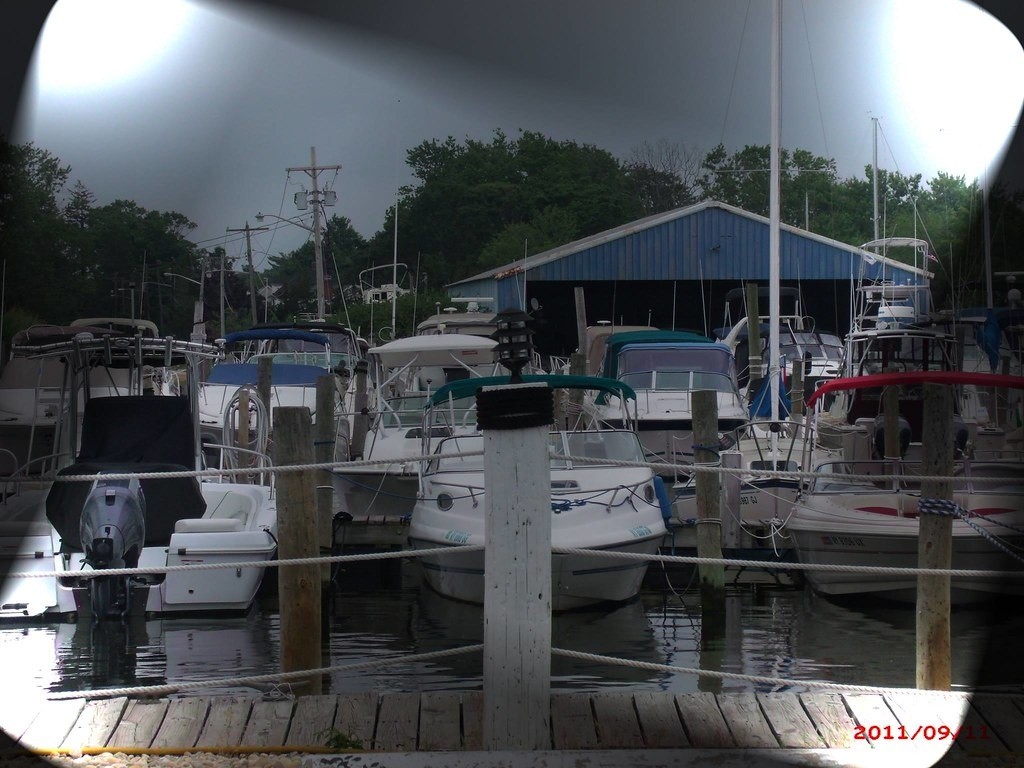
[163, 273, 204, 324]
[118, 288, 149, 318]
[255, 214, 325, 319]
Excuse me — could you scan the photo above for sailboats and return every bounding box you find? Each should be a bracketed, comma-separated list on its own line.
[826, 110, 958, 399]
[668, 1, 896, 594]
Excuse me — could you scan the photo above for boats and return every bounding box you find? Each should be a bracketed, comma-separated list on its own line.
[0, 198, 755, 623]
[783, 238, 1024, 603]
